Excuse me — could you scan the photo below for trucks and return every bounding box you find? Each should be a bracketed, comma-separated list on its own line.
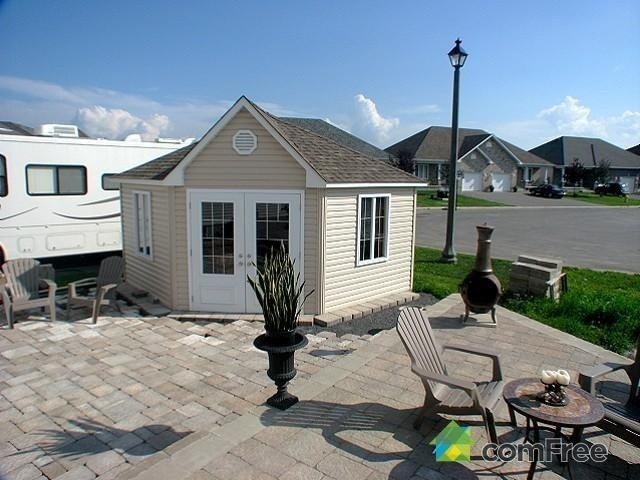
[593, 177, 639, 195]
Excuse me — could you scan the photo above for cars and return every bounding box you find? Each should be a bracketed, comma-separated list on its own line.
[529, 183, 567, 199]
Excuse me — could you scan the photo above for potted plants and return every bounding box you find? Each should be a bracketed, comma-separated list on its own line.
[247, 239, 315, 410]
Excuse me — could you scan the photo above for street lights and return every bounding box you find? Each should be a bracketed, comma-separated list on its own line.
[438, 38, 468, 263]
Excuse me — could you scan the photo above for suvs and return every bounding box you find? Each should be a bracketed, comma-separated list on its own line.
[594, 181, 630, 197]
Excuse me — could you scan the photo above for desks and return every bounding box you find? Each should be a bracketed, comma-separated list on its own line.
[503, 378, 605, 480]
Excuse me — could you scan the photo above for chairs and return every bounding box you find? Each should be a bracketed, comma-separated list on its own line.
[65, 256, 125, 324]
[396, 306, 517, 455]
[0, 259, 57, 329]
[570, 342, 640, 462]
